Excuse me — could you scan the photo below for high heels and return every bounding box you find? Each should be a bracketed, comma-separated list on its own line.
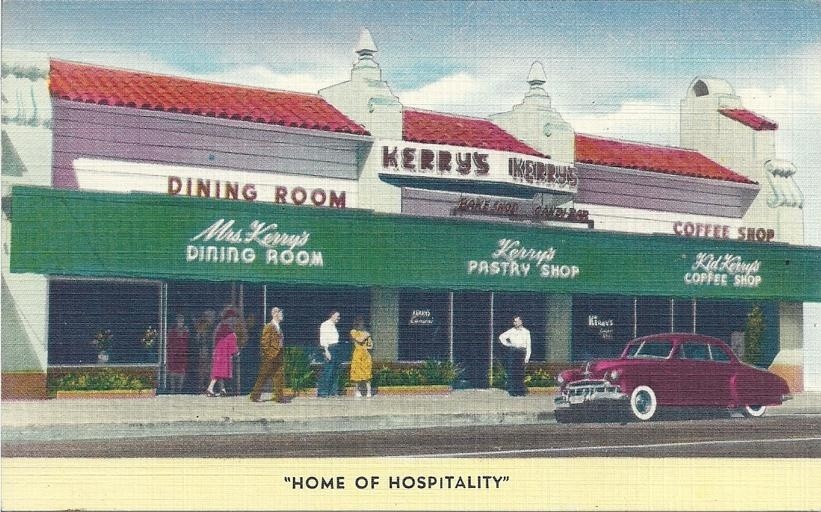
[203, 390, 218, 398]
[220, 391, 228, 397]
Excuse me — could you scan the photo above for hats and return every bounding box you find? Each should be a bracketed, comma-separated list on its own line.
[270, 307, 285, 315]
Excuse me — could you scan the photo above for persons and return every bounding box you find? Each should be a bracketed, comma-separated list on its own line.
[314, 309, 343, 398]
[348, 316, 375, 398]
[252, 307, 291, 405]
[167, 305, 248, 397]
[498, 313, 533, 399]
